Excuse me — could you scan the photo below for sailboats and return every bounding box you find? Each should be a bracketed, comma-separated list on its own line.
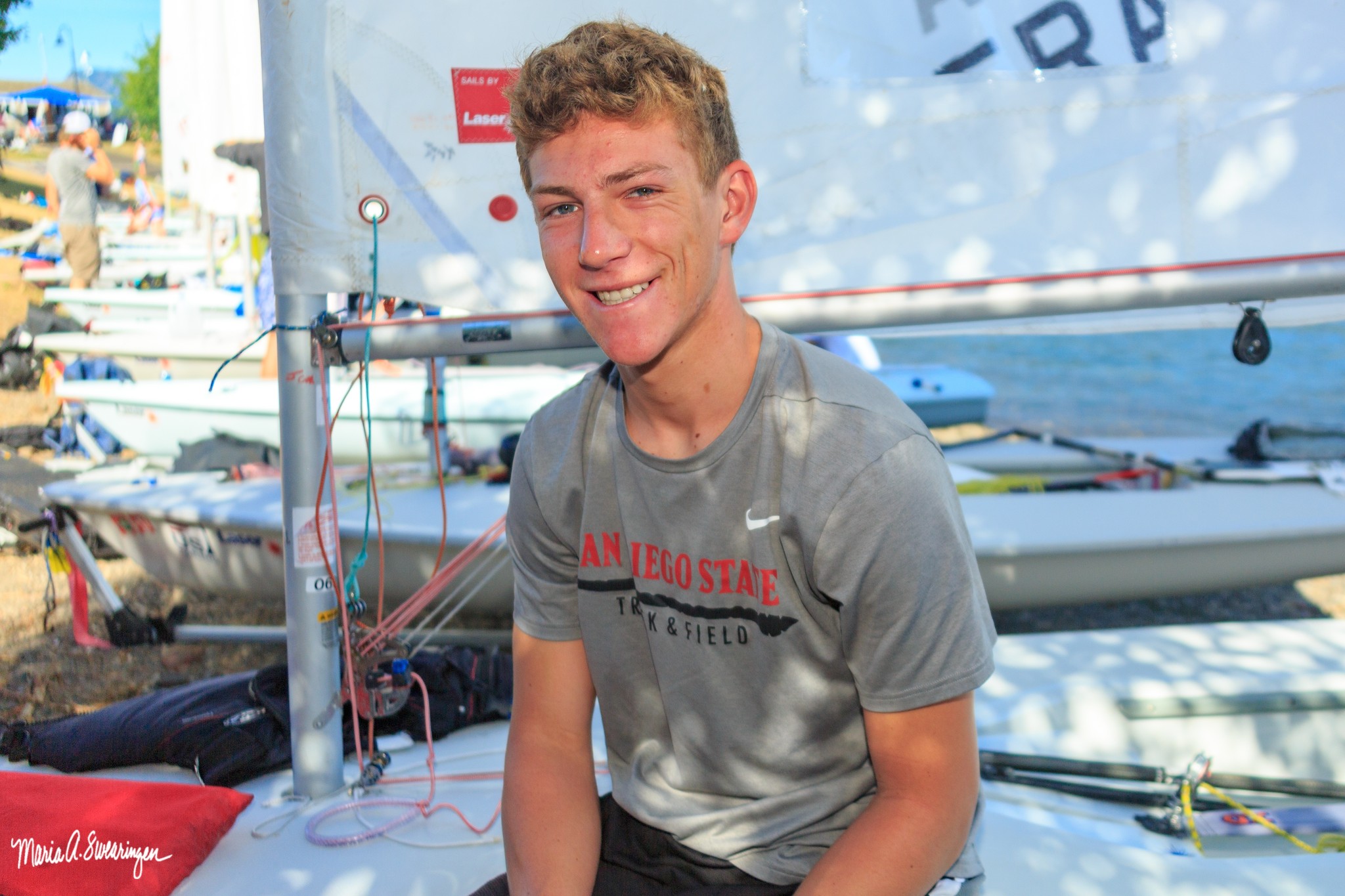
[16, 1, 1345, 629]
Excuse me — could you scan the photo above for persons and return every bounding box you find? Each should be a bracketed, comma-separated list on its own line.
[468, 22, 999, 896]
[45, 108, 119, 289]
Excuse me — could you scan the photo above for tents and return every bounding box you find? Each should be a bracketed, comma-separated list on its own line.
[0, 84, 119, 142]
